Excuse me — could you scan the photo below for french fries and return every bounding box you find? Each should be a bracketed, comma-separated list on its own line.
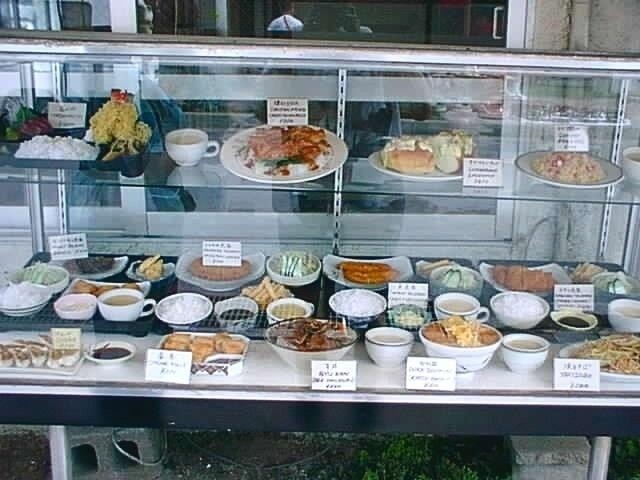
[251, 277, 288, 306]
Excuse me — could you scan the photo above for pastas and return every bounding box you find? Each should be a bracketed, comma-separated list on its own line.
[571, 335, 640, 375]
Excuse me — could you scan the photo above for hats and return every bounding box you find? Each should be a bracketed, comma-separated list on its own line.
[267, 14, 304, 32]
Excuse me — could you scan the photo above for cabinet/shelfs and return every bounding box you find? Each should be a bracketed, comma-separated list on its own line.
[0, 29, 640, 480]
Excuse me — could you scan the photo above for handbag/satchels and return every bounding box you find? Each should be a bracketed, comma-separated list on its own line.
[179, 186, 197, 212]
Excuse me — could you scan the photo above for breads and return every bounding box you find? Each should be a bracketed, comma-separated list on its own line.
[381, 128, 477, 173]
[163, 334, 245, 364]
[492, 266, 558, 291]
[71, 281, 140, 296]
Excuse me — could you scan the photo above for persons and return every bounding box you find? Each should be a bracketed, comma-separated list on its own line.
[56, 53, 191, 396]
[256, 0, 424, 421]
[337, 7, 372, 37]
[266, 0, 305, 31]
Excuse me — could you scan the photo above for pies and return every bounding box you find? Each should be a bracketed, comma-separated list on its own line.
[336, 261, 398, 284]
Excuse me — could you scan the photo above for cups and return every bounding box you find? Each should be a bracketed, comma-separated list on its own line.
[623, 143, 640, 186]
[161, 128, 220, 167]
[166, 166, 222, 187]
[433, 292, 490, 322]
[98, 287, 157, 322]
[363, 326, 415, 368]
[502, 333, 551, 373]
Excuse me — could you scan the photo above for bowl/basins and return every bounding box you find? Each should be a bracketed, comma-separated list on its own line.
[550, 307, 599, 331]
[386, 304, 430, 330]
[265, 297, 314, 324]
[215, 296, 258, 329]
[54, 292, 98, 321]
[327, 289, 388, 328]
[491, 290, 550, 330]
[607, 298, 640, 333]
[419, 318, 502, 371]
[1, 283, 53, 318]
[265, 318, 358, 372]
[84, 340, 136, 365]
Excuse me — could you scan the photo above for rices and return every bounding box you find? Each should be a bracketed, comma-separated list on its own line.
[160, 295, 209, 322]
[493, 294, 544, 317]
[332, 290, 383, 316]
[0, 280, 49, 308]
[532, 152, 606, 184]
[13, 134, 101, 161]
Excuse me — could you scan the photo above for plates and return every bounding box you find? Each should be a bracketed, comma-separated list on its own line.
[265, 250, 322, 287]
[367, 150, 482, 182]
[0, 339, 92, 377]
[155, 292, 214, 325]
[515, 146, 626, 191]
[61, 255, 128, 279]
[415, 258, 484, 289]
[6, 263, 70, 295]
[125, 260, 175, 283]
[322, 252, 414, 288]
[174, 249, 267, 291]
[479, 261, 573, 296]
[157, 331, 250, 372]
[1, 249, 638, 296]
[219, 121, 350, 187]
[560, 341, 640, 382]
[590, 272, 639, 295]
